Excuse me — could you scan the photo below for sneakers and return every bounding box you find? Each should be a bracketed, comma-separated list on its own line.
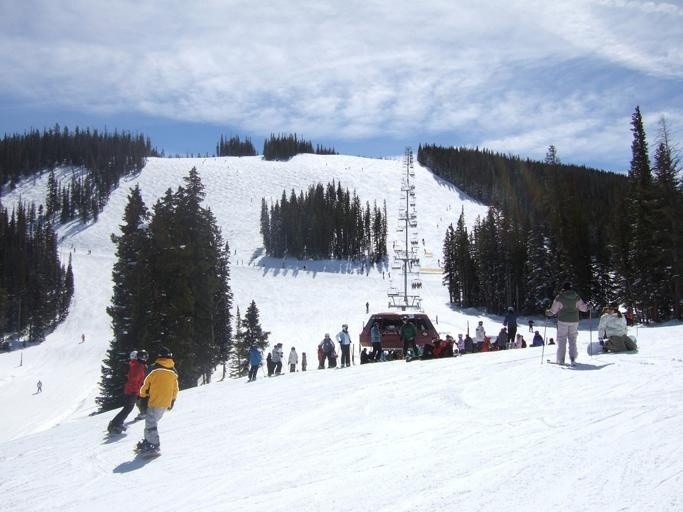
[136, 438, 148, 449]
[107, 420, 127, 431]
[137, 412, 147, 419]
[141, 443, 160, 452]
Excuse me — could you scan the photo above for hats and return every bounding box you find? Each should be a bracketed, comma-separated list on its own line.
[609, 301, 619, 310]
[157, 346, 172, 358]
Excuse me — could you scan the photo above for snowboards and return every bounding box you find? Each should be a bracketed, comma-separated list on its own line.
[587, 335, 637, 355]
[134, 444, 160, 459]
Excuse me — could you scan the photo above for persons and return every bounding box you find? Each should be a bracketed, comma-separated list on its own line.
[137, 345, 179, 452]
[109, 349, 149, 431]
[248, 344, 262, 382]
[267, 343, 307, 377]
[361, 306, 555, 364]
[129, 350, 148, 421]
[598, 303, 637, 353]
[318, 324, 351, 369]
[544, 282, 589, 367]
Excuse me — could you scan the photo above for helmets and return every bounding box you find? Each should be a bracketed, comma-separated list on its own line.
[136, 349, 150, 361]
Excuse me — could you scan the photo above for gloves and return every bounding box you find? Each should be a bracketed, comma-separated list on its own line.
[545, 309, 554, 317]
[586, 301, 594, 310]
[168, 400, 174, 410]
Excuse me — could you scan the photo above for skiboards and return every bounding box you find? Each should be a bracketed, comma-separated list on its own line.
[547, 360, 578, 369]
